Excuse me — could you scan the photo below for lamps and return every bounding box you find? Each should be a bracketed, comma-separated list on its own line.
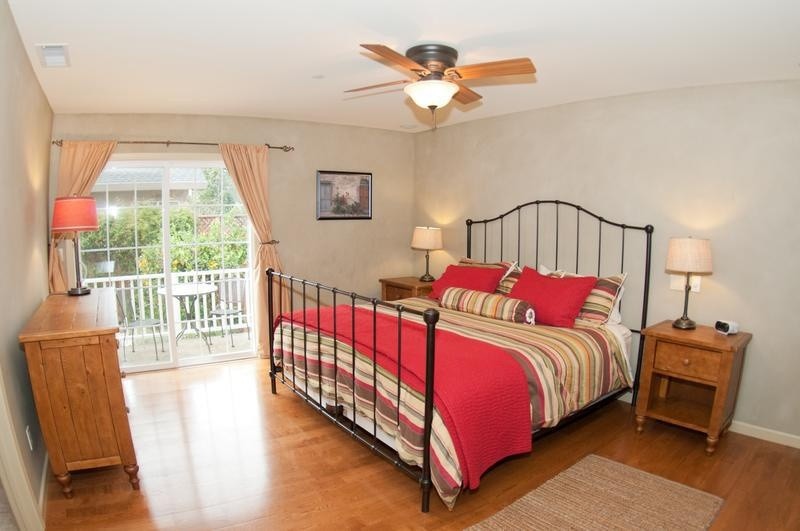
[411, 226, 443, 282]
[666, 234, 713, 330]
[52, 192, 99, 296]
[404, 77, 460, 115]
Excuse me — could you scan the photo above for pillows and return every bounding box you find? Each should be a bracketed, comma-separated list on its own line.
[429, 257, 628, 327]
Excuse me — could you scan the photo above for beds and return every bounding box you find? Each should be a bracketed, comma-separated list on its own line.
[265, 198, 654, 512]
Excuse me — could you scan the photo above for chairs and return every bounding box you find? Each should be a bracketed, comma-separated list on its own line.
[208, 279, 250, 347]
[116, 289, 165, 364]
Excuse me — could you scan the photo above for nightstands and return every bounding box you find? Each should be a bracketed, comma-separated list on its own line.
[379, 277, 436, 302]
[635, 320, 753, 457]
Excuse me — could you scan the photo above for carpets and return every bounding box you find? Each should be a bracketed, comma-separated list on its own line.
[461, 454, 724, 531]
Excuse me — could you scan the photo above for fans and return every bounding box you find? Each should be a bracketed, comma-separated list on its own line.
[344, 44, 537, 106]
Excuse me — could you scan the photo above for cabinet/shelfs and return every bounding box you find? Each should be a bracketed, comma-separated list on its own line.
[19, 287, 139, 500]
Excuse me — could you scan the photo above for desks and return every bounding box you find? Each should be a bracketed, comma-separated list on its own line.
[157, 283, 219, 353]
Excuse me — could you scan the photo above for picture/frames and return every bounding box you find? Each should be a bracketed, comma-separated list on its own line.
[316, 171, 373, 220]
